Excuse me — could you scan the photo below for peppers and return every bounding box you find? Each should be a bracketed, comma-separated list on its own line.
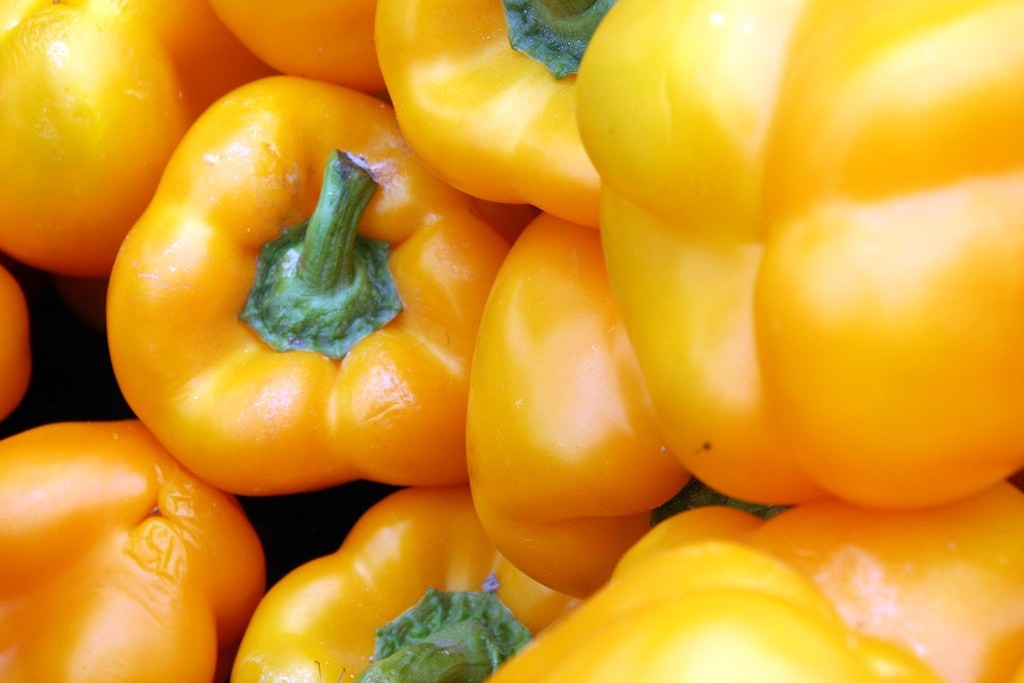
[0, 0, 1024, 683]
